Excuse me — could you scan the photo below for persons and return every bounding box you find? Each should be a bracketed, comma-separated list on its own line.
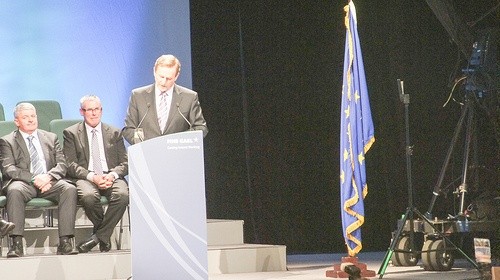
[123, 54, 208, 144]
[0, 102, 78, 257]
[62, 95, 129, 253]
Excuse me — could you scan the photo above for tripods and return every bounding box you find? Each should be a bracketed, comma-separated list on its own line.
[378, 80, 480, 279]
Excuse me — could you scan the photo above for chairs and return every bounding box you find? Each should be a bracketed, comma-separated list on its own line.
[0, 100, 124, 258]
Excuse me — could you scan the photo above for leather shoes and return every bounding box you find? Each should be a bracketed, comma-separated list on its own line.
[6, 241, 23, 257]
[99, 238, 111, 253]
[56, 240, 80, 255]
[77, 235, 99, 253]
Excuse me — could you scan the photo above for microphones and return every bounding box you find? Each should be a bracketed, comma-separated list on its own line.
[176, 103, 192, 131]
[136, 102, 151, 142]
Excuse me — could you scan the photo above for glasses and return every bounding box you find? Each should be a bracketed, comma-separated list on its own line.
[82, 107, 102, 113]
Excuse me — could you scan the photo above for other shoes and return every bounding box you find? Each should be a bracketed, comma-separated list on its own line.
[0, 220, 15, 238]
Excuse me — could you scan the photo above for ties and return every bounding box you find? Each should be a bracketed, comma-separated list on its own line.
[157, 92, 168, 134]
[91, 129, 104, 176]
[27, 135, 45, 176]
